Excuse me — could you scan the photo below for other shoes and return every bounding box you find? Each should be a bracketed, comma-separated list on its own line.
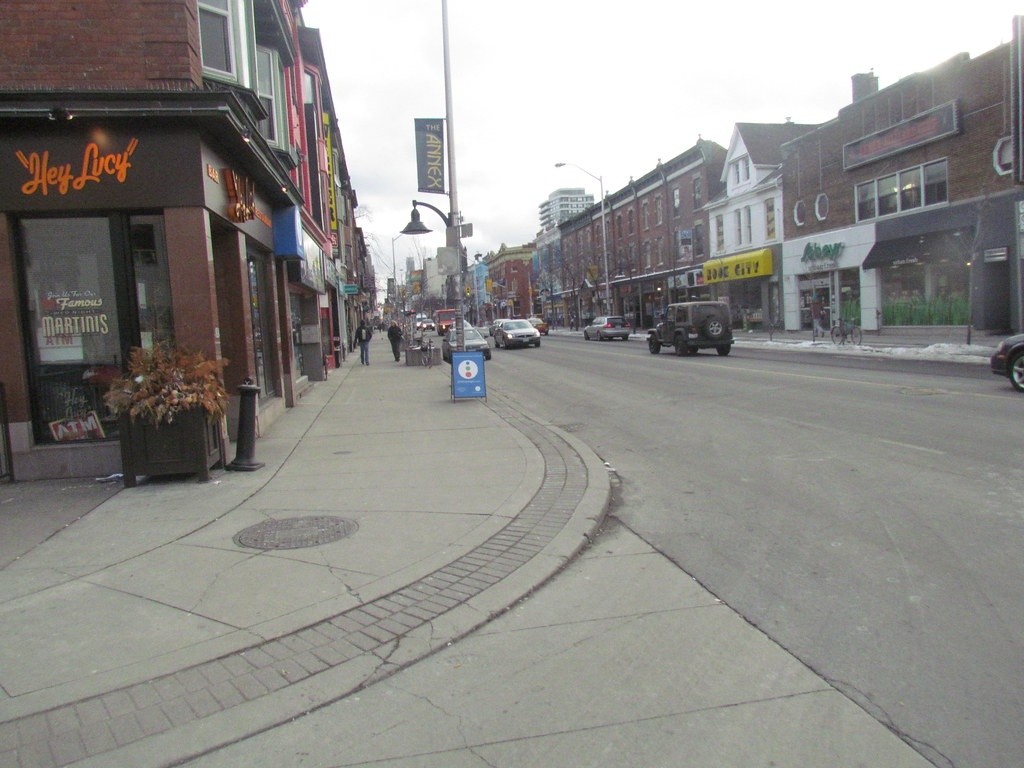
[362, 362, 369, 365]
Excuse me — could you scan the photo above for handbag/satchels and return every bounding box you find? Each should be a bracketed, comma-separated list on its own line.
[401, 335, 405, 342]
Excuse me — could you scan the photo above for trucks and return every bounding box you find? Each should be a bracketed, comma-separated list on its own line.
[434, 309, 456, 336]
[416, 314, 427, 330]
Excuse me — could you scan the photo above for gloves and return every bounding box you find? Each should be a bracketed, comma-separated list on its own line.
[353, 343, 356, 348]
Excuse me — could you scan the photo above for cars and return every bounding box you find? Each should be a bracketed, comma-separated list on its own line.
[493, 319, 540, 349]
[527, 318, 549, 335]
[489, 319, 512, 336]
[421, 319, 435, 332]
[447, 320, 473, 332]
[990, 333, 1024, 393]
[442, 328, 491, 363]
[584, 316, 631, 341]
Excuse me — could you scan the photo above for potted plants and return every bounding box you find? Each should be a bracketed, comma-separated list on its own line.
[103, 343, 230, 487]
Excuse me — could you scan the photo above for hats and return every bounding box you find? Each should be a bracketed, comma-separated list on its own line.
[360, 320, 365, 325]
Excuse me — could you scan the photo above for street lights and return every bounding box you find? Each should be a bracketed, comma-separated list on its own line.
[399, 200, 468, 319]
[392, 234, 404, 306]
[459, 251, 481, 327]
[492, 282, 510, 318]
[555, 162, 611, 315]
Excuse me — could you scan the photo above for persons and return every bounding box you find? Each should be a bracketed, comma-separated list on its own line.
[354, 320, 372, 365]
[567, 312, 576, 332]
[810, 296, 825, 338]
[387, 320, 402, 363]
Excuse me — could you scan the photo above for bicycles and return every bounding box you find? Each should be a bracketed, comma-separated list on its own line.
[831, 317, 862, 346]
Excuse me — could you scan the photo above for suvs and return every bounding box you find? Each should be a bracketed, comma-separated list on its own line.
[645, 300, 735, 356]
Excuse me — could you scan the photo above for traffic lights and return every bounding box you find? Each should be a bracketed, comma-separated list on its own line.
[465, 287, 471, 297]
